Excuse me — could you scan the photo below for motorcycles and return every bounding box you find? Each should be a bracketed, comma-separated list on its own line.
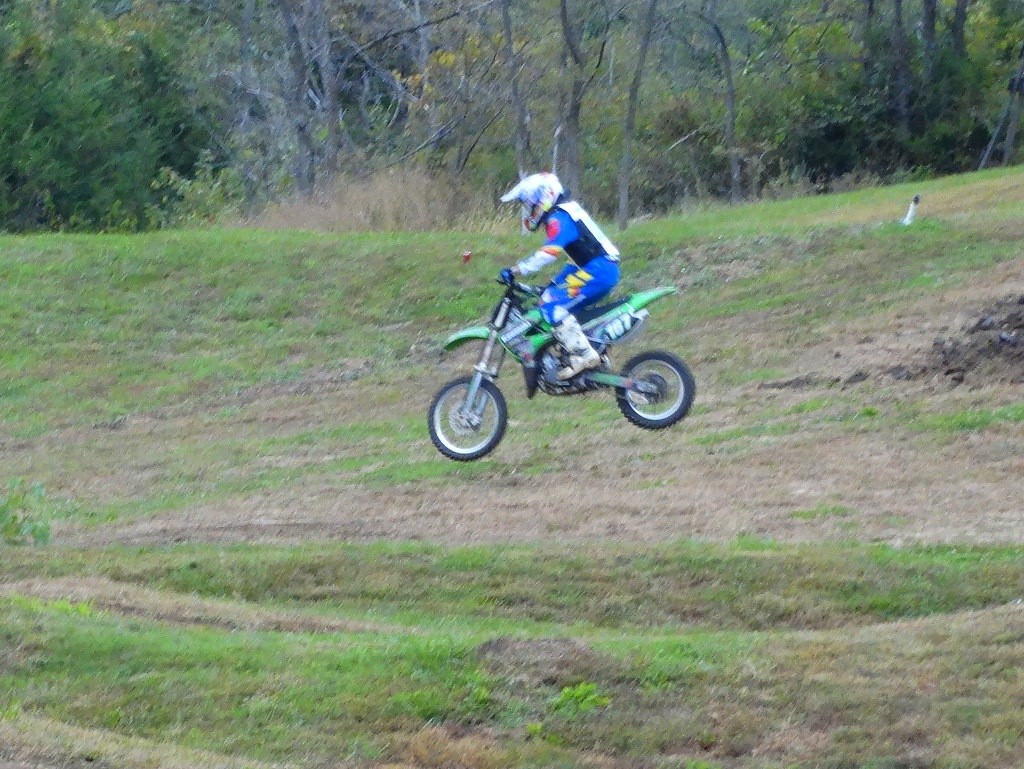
[428, 268, 696, 462]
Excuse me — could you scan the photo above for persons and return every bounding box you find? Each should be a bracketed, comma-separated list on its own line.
[496, 172, 619, 380]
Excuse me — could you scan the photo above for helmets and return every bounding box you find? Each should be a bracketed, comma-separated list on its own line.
[500, 174, 571, 231]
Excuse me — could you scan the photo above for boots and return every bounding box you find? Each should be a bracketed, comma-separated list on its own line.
[551, 313, 601, 380]
